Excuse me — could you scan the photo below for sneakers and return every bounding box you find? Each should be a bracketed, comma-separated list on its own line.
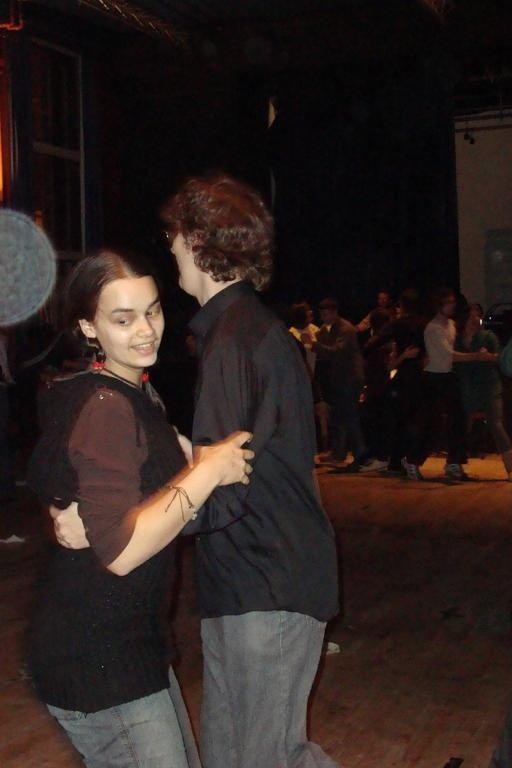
[318, 453, 346, 465]
[404, 463, 422, 479]
[444, 463, 469, 481]
[356, 458, 390, 472]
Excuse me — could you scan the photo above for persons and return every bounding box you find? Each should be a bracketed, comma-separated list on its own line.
[48, 173, 348, 768]
[181, 334, 199, 384]
[288, 286, 510, 483]
[23, 248, 258, 768]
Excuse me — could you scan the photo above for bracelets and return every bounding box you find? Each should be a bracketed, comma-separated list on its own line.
[166, 485, 200, 524]
